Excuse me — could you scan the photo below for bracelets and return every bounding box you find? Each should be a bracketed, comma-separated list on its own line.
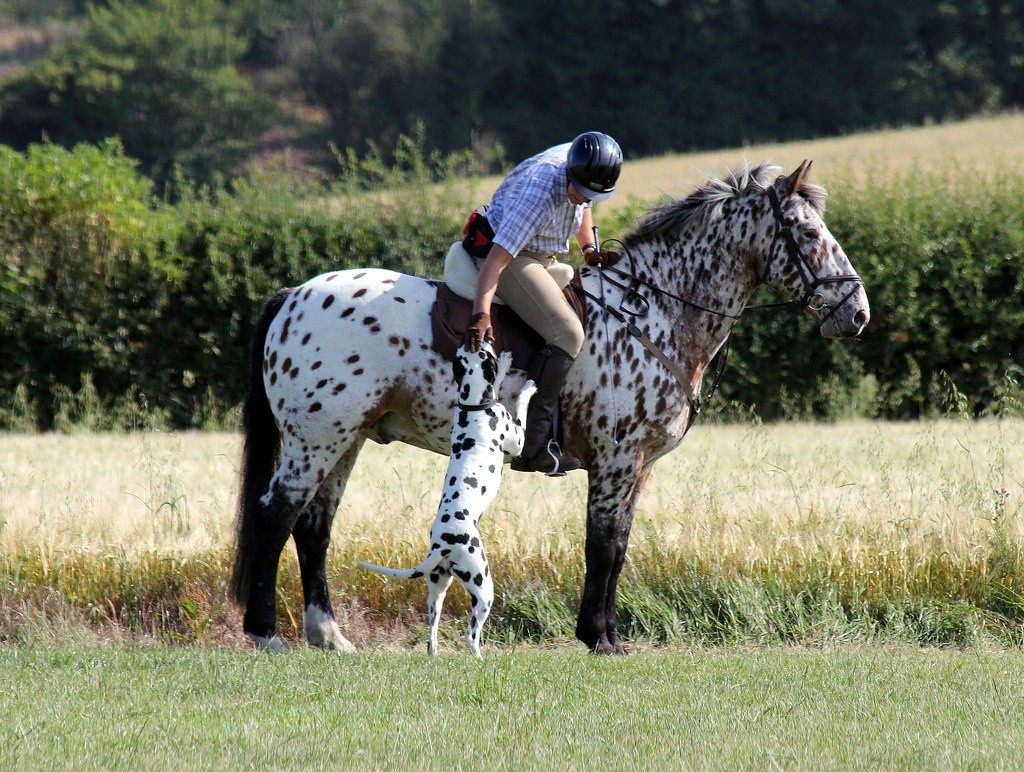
[579, 242, 596, 256]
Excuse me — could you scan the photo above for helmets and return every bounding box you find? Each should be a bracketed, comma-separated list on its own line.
[567, 131, 622, 202]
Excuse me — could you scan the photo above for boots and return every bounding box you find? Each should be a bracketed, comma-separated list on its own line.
[510, 343, 582, 473]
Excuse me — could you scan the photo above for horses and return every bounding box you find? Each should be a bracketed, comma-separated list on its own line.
[228, 156, 872, 655]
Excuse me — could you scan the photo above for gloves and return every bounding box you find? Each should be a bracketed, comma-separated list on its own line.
[464, 312, 495, 353]
[585, 248, 620, 271]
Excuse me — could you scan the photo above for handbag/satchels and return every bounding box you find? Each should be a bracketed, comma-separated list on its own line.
[462, 204, 497, 259]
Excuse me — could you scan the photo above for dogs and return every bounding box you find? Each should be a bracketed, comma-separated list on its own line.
[357, 338, 539, 663]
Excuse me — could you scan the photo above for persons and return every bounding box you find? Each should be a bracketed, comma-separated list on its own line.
[461, 130, 624, 477]
